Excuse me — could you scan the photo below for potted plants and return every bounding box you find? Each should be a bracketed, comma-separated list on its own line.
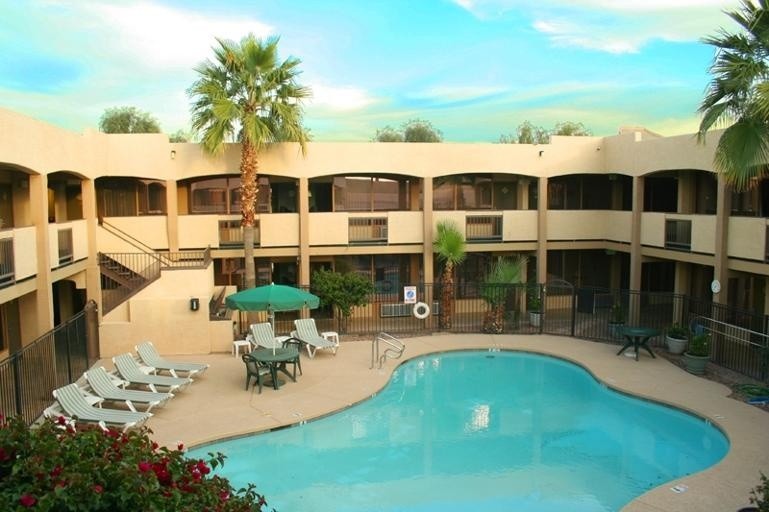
[682, 325, 713, 376]
[607, 299, 627, 340]
[664, 318, 690, 356]
[524, 281, 547, 328]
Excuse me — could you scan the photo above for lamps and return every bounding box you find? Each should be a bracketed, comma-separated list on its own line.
[191, 297, 199, 311]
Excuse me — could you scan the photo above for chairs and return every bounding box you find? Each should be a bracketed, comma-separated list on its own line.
[42, 340, 211, 443]
[231, 318, 340, 395]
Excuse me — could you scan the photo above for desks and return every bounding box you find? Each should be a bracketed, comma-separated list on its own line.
[613, 325, 663, 362]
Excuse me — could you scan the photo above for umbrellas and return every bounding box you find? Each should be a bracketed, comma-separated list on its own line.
[224, 280, 321, 357]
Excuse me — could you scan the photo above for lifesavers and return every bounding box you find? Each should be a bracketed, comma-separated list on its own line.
[413, 302, 430, 319]
